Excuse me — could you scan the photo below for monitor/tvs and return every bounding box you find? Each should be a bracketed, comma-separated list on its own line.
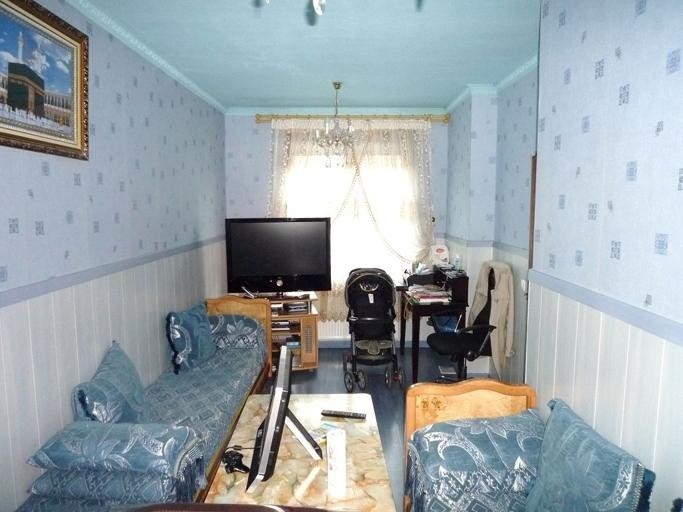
[225, 217, 332, 300]
[243, 344, 324, 495]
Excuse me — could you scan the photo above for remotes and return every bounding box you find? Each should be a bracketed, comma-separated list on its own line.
[297, 293, 310, 300]
[320, 409, 367, 419]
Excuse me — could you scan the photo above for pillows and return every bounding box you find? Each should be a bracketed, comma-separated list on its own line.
[407, 408, 547, 495]
[25, 421, 207, 479]
[166, 303, 262, 374]
[31, 470, 176, 502]
[424, 492, 526, 512]
[73, 344, 142, 424]
[525, 399, 645, 512]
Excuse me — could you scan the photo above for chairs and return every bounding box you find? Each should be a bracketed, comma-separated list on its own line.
[427, 269, 497, 382]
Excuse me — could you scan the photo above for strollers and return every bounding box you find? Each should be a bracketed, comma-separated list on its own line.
[340, 267, 404, 394]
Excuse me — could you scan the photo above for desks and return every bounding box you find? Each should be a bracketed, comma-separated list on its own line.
[399, 268, 469, 384]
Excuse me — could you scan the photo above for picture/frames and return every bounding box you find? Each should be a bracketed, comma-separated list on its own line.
[0, 0, 89, 160]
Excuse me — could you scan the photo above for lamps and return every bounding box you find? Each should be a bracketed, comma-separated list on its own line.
[314, 82, 358, 168]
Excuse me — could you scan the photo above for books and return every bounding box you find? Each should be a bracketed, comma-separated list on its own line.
[420, 295, 449, 303]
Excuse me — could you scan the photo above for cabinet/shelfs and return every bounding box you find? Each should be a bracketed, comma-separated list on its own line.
[236, 291, 319, 372]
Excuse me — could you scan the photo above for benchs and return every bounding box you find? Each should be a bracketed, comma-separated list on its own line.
[402, 377, 537, 512]
[14, 295, 272, 512]
